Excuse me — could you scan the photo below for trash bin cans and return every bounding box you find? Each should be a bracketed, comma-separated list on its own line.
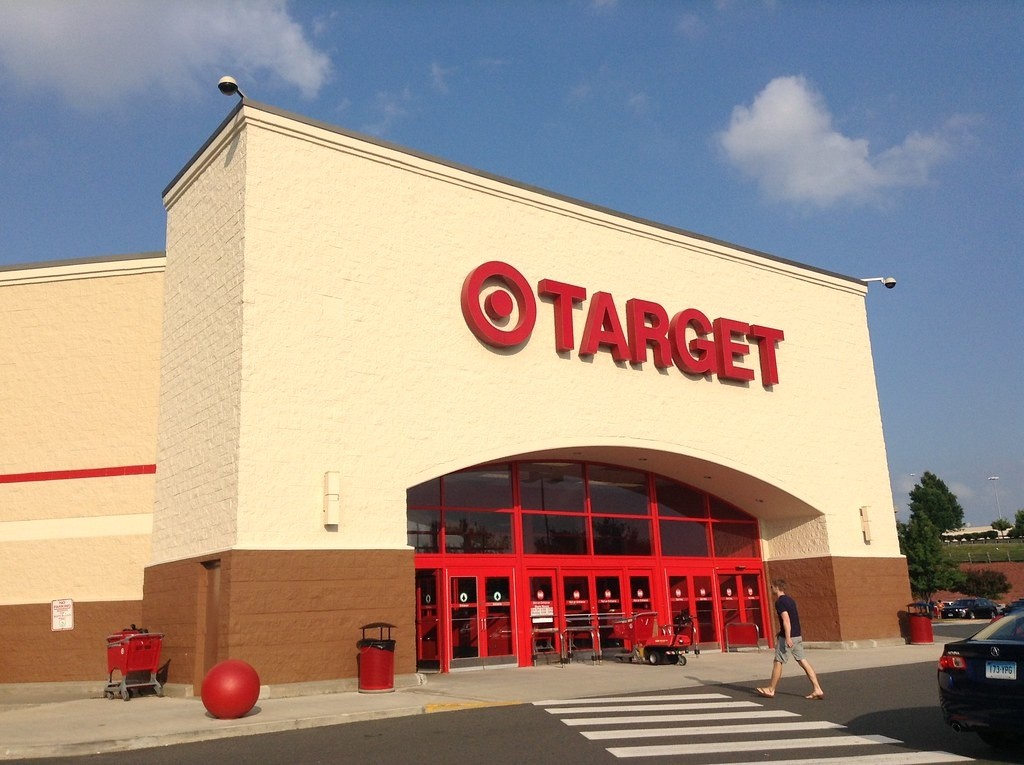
[905, 602, 934, 645]
[358, 622, 398, 694]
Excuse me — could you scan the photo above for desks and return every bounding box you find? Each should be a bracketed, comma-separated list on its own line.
[531, 628, 564, 666]
[562, 626, 602, 667]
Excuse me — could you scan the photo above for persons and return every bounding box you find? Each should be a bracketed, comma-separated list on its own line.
[937, 599, 945, 618]
[754, 577, 824, 700]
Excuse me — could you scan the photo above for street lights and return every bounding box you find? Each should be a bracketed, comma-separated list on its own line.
[988, 477, 1002, 519]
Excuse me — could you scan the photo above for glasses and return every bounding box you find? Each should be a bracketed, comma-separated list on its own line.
[771, 586, 773, 588]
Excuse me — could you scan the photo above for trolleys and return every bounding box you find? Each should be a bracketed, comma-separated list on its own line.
[105, 631, 165, 701]
[610, 611, 658, 663]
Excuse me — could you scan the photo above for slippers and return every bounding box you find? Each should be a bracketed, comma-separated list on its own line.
[806, 693, 825, 699]
[755, 687, 775, 697]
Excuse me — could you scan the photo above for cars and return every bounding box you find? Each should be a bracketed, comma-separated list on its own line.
[940, 598, 998, 619]
[991, 601, 1006, 614]
[932, 602, 954, 612]
[937, 608, 1024, 746]
[999, 600, 1024, 616]
